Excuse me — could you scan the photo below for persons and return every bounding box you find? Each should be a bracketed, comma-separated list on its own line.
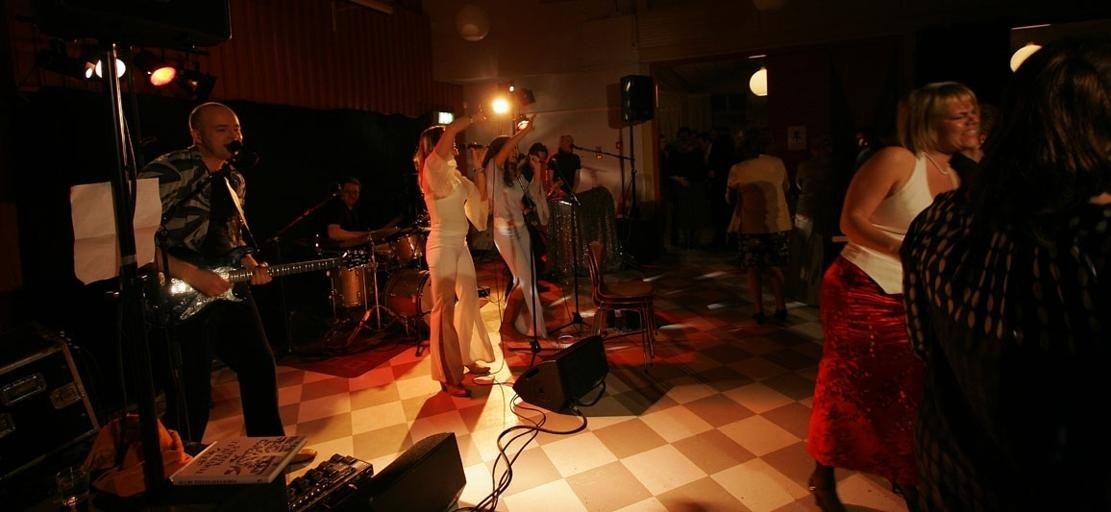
[950, 142, 985, 189]
[722, 127, 800, 327]
[325, 175, 375, 243]
[804, 80, 982, 509]
[481, 134, 551, 343]
[412, 125, 492, 398]
[896, 41, 1111, 510]
[545, 134, 581, 197]
[517, 141, 553, 293]
[136, 100, 318, 464]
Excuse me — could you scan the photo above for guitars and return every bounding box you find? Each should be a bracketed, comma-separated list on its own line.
[168, 248, 366, 322]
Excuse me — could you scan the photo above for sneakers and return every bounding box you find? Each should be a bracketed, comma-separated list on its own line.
[292, 449, 317, 463]
[754, 312, 764, 325]
[774, 309, 788, 319]
[537, 283, 550, 293]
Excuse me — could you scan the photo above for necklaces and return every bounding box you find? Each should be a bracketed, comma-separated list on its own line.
[921, 151, 953, 179]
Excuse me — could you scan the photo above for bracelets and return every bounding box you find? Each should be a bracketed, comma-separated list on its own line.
[467, 114, 478, 125]
[506, 138, 520, 149]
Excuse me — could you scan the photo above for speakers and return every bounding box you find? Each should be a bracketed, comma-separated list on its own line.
[226, 140, 261, 167]
[514, 333, 610, 411]
[620, 73, 657, 122]
[359, 432, 466, 512]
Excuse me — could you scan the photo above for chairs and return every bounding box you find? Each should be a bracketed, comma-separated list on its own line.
[584, 241, 661, 370]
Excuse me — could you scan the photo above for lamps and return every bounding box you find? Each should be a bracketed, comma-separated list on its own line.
[134, 51, 176, 87]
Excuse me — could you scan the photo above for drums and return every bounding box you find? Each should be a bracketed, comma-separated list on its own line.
[391, 229, 425, 264]
[384, 269, 434, 340]
[331, 265, 370, 308]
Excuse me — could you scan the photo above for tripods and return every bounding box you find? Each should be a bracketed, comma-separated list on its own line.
[502, 207, 567, 375]
[617, 126, 662, 239]
[553, 196, 605, 334]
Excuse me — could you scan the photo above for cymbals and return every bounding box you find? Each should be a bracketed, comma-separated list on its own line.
[341, 226, 400, 247]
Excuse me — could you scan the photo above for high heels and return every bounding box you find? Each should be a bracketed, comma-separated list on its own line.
[808, 471, 847, 512]
[499, 329, 527, 342]
[439, 379, 471, 397]
[891, 481, 920, 512]
[463, 362, 490, 374]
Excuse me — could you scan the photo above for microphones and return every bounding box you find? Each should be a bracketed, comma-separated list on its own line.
[547, 153, 562, 172]
[519, 154, 541, 163]
[457, 143, 484, 150]
[572, 143, 581, 150]
